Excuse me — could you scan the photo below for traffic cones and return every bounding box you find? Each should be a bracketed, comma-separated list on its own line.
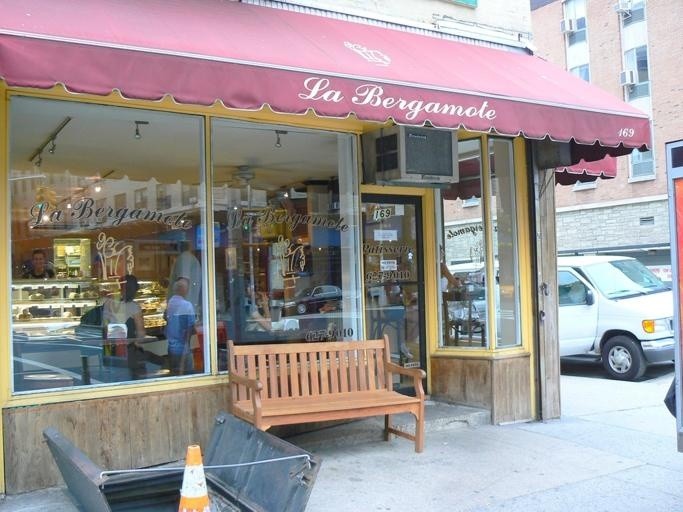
[173, 439, 215, 512]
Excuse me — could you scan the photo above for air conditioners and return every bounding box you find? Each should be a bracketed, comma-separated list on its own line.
[361, 124, 459, 187]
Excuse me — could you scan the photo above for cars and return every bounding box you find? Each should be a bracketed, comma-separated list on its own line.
[281, 281, 345, 318]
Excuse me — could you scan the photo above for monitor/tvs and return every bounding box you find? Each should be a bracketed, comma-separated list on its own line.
[195, 222, 221, 251]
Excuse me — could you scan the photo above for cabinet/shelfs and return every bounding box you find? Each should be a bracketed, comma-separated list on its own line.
[11, 279, 167, 338]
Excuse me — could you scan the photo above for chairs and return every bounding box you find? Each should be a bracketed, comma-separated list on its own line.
[441, 289, 486, 347]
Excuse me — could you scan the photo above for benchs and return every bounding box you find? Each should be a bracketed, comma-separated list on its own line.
[228, 334, 429, 458]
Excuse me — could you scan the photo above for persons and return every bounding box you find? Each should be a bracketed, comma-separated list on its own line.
[163, 279, 199, 376]
[168, 240, 201, 305]
[439, 244, 460, 287]
[566, 280, 586, 304]
[388, 280, 401, 295]
[17, 249, 58, 279]
[99, 275, 148, 379]
[227, 272, 272, 332]
[449, 277, 479, 321]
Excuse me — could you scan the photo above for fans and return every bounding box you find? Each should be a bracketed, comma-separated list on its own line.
[189, 164, 294, 197]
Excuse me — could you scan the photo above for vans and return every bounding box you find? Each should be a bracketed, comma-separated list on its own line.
[447, 253, 676, 383]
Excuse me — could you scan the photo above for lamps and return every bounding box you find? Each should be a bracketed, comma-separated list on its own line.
[26, 169, 117, 229]
[131, 119, 149, 138]
[27, 116, 72, 168]
[275, 128, 287, 149]
